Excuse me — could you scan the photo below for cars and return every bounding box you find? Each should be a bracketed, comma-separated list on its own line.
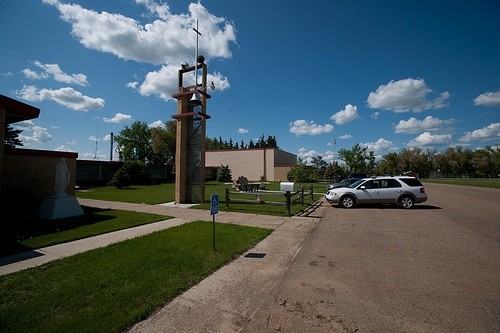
[328, 177, 360, 191]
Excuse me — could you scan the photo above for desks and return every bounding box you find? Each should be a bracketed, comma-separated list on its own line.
[224, 181, 270, 192]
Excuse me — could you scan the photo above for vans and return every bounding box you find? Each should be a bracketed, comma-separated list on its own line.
[325, 175, 428, 209]
